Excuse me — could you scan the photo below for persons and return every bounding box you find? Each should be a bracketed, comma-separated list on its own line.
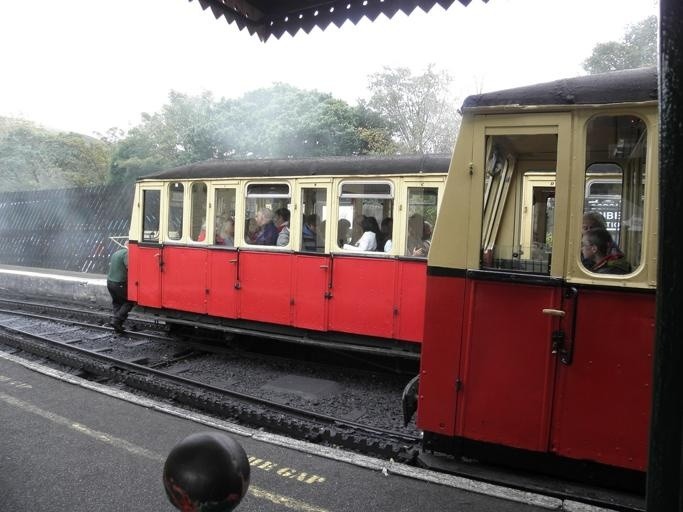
[581, 211, 632, 274]
[106, 239, 134, 333]
[196, 206, 433, 259]
[580, 228, 626, 275]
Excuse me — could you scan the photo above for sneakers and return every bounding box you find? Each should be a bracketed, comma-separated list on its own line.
[109, 320, 125, 333]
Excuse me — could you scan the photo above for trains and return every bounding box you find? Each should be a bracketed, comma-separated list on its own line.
[399, 62, 660, 488]
[123, 151, 624, 376]
